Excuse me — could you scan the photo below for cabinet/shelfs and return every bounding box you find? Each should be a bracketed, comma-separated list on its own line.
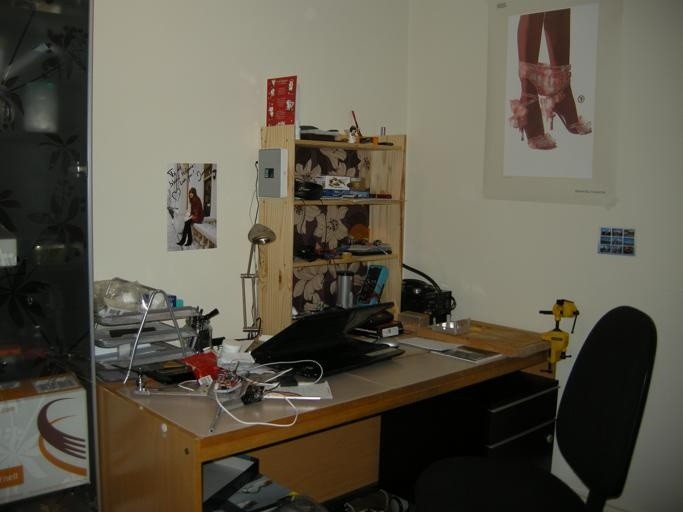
[0, 1, 93, 512]
[259, 125, 408, 336]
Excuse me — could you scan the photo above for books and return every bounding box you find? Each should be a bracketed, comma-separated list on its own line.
[261, 381, 334, 400]
[428, 344, 502, 364]
[398, 337, 465, 352]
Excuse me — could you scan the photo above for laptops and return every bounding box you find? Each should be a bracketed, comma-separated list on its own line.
[251, 301, 404, 377]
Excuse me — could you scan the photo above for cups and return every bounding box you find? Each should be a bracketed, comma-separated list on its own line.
[336, 271, 353, 309]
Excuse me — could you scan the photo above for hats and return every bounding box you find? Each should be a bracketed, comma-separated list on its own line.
[188, 188, 196, 195]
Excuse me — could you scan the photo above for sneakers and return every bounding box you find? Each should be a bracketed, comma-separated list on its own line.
[176, 241, 192, 246]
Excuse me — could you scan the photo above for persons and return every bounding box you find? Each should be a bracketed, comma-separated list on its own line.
[175, 186, 205, 247]
[511, 10, 593, 151]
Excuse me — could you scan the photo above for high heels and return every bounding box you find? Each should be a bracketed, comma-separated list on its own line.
[547, 86, 594, 133]
[513, 93, 555, 150]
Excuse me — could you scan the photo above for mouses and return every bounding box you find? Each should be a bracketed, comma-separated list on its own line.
[372, 337, 400, 348]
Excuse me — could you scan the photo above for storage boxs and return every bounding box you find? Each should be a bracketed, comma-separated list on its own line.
[0, 372, 91, 507]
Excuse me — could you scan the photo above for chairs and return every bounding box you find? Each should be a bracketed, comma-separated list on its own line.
[416, 305, 658, 511]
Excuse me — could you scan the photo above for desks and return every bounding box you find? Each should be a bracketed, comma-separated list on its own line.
[96, 312, 552, 510]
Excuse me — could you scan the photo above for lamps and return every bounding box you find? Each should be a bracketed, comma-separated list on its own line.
[239, 223, 276, 339]
